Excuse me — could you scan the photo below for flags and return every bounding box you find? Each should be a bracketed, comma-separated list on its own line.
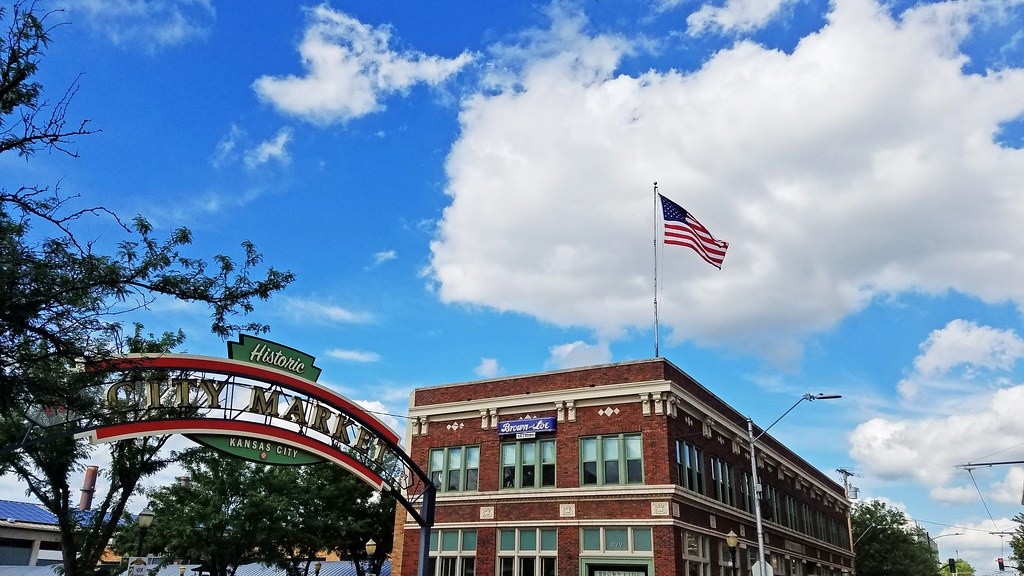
[658, 193, 729, 269]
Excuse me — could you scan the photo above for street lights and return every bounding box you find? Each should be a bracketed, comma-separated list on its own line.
[850, 523, 876, 552]
[927, 531, 964, 557]
[179, 566, 186, 576]
[746, 392, 843, 576]
[135, 507, 155, 556]
[365, 538, 376, 575]
[725, 528, 738, 576]
[315, 561, 322, 576]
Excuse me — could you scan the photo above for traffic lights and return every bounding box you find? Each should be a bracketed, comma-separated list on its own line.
[998, 558, 1005, 571]
